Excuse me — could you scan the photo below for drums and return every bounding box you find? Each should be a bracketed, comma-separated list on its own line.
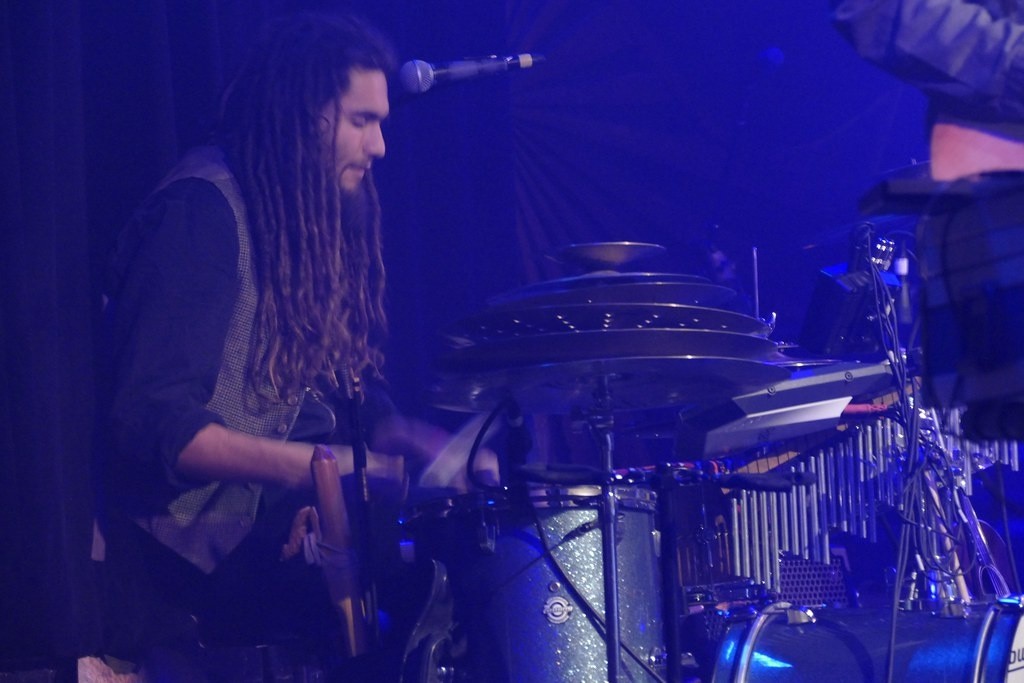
[383, 482, 672, 683]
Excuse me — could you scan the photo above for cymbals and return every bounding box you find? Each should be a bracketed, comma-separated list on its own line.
[447, 241, 792, 417]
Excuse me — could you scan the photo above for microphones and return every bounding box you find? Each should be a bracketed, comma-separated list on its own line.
[400, 53, 546, 97]
[895, 241, 915, 325]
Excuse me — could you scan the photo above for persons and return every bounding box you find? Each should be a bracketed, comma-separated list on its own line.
[92, 10, 500, 683]
